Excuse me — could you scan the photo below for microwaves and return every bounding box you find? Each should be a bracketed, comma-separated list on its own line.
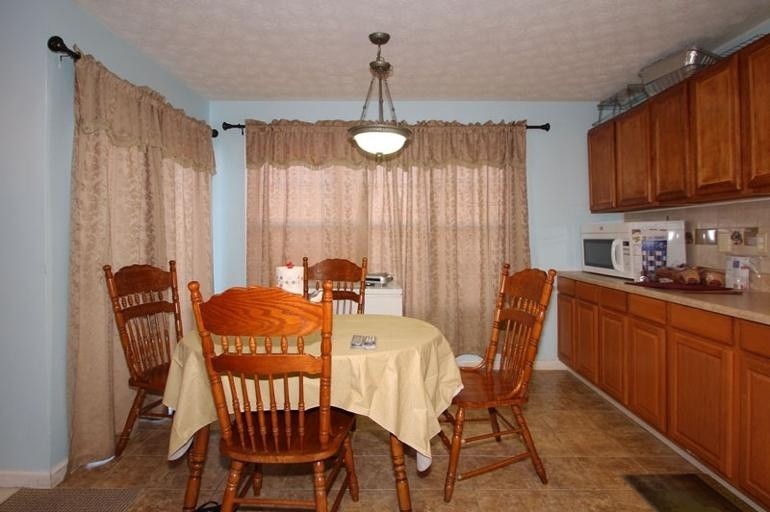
[579, 220, 687, 280]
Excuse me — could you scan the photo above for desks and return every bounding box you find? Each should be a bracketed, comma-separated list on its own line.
[278, 281, 403, 315]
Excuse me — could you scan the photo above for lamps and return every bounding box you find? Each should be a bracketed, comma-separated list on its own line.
[346, 32, 412, 166]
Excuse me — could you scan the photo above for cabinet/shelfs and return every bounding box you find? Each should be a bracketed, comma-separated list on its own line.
[599, 286, 669, 432]
[651, 51, 740, 206]
[587, 95, 651, 213]
[740, 33, 770, 200]
[669, 299, 770, 512]
[557, 274, 599, 386]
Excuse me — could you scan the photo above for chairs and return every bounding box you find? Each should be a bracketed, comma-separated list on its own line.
[186, 280, 362, 512]
[301, 254, 367, 315]
[427, 260, 557, 503]
[102, 259, 204, 457]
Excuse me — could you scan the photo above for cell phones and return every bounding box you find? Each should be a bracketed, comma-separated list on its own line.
[364, 336, 376, 347]
[351, 335, 363, 347]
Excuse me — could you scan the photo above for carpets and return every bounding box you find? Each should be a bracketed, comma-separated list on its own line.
[1, 484, 142, 512]
[623, 470, 745, 512]
